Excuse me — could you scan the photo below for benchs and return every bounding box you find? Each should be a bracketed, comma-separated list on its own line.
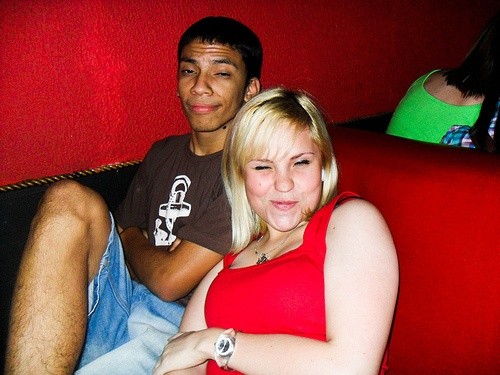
[328, 127, 500, 375]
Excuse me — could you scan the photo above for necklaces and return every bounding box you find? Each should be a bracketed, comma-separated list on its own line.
[255, 231, 292, 265]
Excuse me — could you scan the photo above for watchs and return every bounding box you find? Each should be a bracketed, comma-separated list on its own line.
[215, 328, 243, 372]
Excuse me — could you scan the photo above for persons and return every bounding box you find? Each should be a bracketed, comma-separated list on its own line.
[5, 14, 264, 375]
[385, 13, 500, 149]
[152, 88, 399, 375]
